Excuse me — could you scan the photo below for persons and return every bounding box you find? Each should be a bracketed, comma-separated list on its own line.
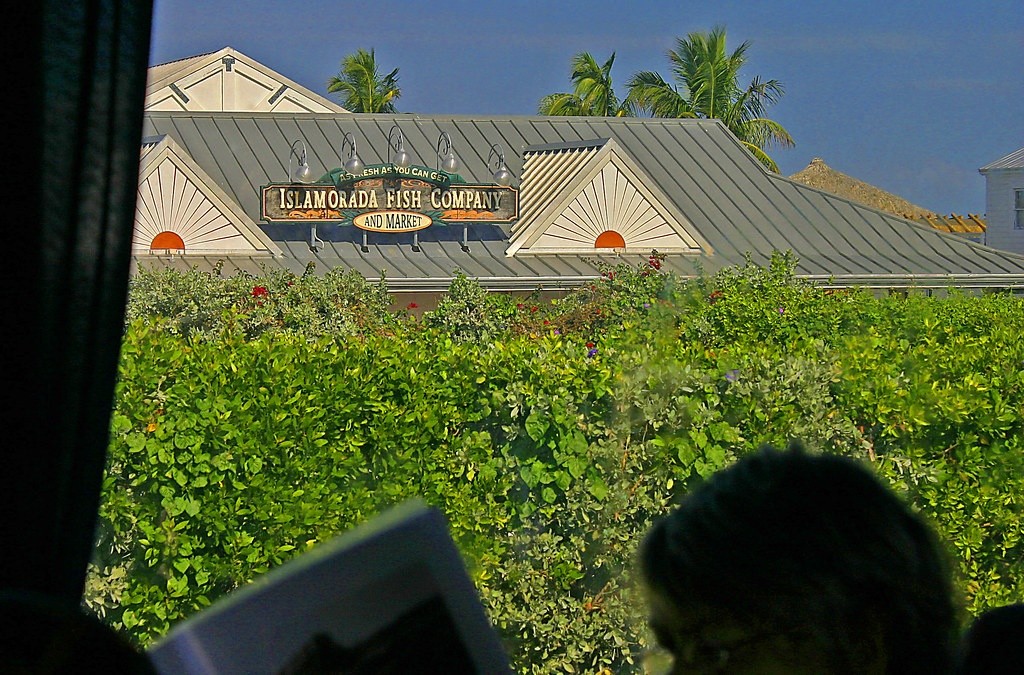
[635, 442, 964, 675]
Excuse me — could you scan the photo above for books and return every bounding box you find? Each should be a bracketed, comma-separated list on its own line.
[149, 499, 511, 675]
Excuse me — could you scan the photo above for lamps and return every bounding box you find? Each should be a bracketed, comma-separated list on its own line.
[289, 140, 312, 184]
[488, 143, 510, 188]
[388, 126, 411, 168]
[341, 132, 364, 174]
[437, 132, 460, 173]
